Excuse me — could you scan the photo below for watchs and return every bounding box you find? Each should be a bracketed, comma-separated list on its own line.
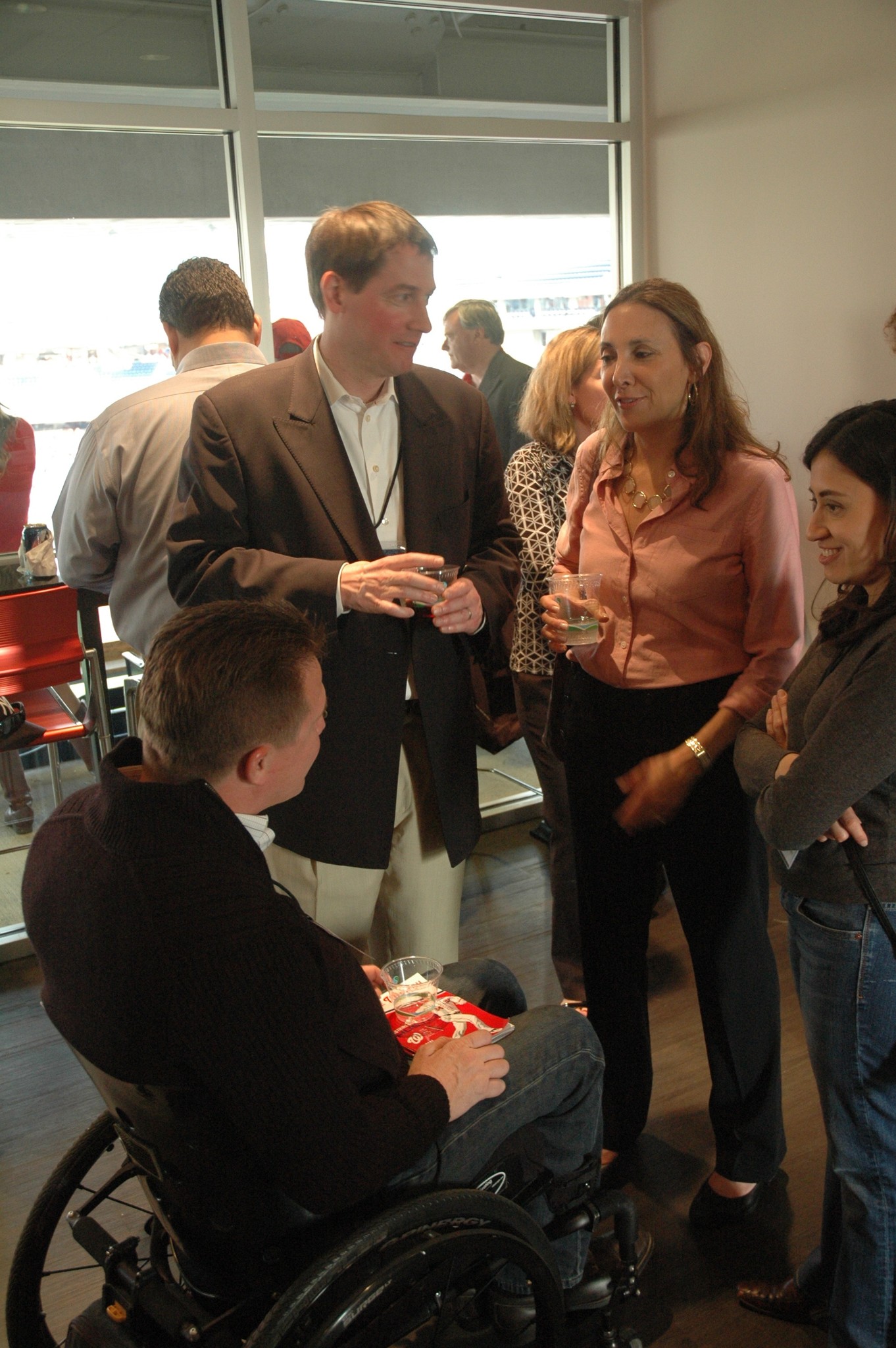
[684, 735, 714, 775]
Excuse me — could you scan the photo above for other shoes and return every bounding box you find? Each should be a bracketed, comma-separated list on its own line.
[689, 1163, 783, 1227]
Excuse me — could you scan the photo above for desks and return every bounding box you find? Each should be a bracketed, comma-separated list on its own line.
[0, 551, 110, 736]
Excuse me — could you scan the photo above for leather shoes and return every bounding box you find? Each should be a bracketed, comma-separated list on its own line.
[452, 1224, 616, 1333]
[734, 1279, 829, 1326]
[489, 1229, 652, 1346]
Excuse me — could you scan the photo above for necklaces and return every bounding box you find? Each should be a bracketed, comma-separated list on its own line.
[619, 441, 685, 513]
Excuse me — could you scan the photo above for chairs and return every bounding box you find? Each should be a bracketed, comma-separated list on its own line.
[0, 586, 111, 809]
[120, 648, 145, 737]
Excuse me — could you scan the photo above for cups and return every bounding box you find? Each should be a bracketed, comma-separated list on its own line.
[545, 574, 603, 645]
[406, 566, 460, 615]
[380, 956, 443, 1024]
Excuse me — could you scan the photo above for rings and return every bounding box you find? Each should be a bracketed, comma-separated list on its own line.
[466, 607, 472, 621]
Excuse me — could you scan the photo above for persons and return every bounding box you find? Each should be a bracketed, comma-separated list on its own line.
[22, 596, 657, 1329]
[541, 277, 809, 1273]
[164, 201, 524, 977]
[48, 255, 293, 668]
[441, 296, 535, 555]
[498, 327, 641, 1013]
[728, 395, 896, 1348]
[272, 317, 313, 364]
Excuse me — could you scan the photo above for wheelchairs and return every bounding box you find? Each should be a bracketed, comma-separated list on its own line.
[0, 948, 647, 1347]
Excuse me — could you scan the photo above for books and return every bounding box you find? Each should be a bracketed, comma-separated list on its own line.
[363, 972, 517, 1070]
